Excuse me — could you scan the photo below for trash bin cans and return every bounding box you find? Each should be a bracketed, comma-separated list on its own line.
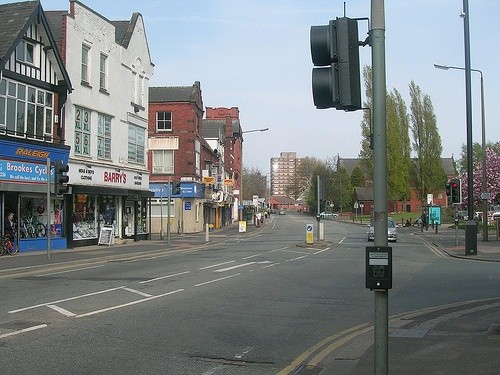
[319, 223, 325, 241]
[261, 216, 263, 223]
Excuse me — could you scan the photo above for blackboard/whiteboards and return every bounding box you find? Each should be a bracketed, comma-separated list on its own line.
[99, 228, 113, 244]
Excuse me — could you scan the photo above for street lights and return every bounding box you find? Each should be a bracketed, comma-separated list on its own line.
[240, 128, 269, 221]
[433, 63, 488, 241]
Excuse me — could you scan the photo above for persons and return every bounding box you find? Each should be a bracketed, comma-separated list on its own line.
[421, 210, 428, 227]
[265, 210, 269, 218]
[256, 210, 264, 227]
[4, 213, 16, 255]
[405, 219, 411, 226]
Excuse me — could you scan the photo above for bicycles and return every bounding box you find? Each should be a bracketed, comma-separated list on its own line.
[0, 211, 47, 256]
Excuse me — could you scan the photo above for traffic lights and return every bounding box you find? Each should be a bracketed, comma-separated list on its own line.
[54, 159, 69, 195]
[172, 179, 180, 195]
[450, 179, 460, 203]
[310, 16, 361, 111]
[446, 183, 452, 197]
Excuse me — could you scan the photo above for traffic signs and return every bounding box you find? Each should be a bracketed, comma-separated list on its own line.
[480, 192, 491, 199]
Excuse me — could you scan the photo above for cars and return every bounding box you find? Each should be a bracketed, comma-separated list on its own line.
[319, 211, 339, 218]
[454, 211, 500, 225]
[279, 209, 285, 215]
[367, 217, 399, 242]
[270, 209, 276, 213]
